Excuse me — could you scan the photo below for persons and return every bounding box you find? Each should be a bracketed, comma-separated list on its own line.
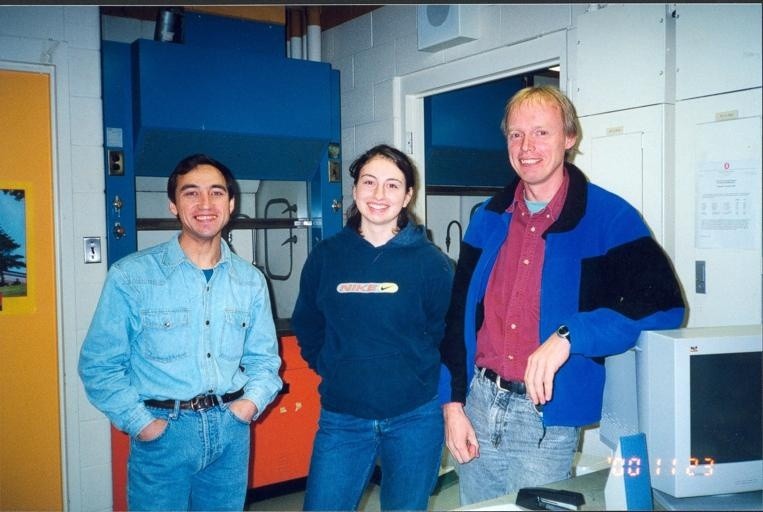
[286, 144, 454, 511]
[76, 155, 284, 510]
[436, 85, 685, 506]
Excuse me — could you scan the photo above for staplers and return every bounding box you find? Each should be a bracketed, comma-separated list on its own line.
[516, 487, 586, 512]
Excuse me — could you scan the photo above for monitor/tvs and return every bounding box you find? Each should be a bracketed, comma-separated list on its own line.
[600, 327, 762, 512]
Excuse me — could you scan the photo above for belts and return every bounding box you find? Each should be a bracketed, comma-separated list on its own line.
[145, 390, 246, 411]
[478, 366, 526, 395]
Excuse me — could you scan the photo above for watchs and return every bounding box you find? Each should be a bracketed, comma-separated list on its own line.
[556, 324, 569, 343]
